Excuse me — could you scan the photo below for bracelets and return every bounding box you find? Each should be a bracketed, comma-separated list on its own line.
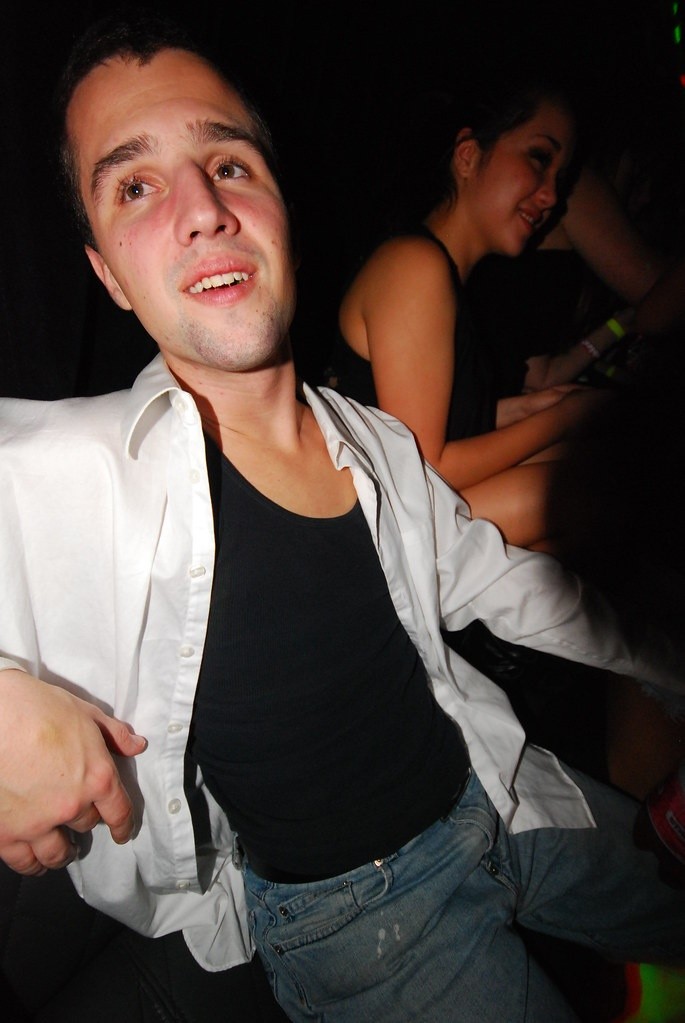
[606, 317, 627, 343]
[604, 365, 624, 387]
[580, 340, 602, 363]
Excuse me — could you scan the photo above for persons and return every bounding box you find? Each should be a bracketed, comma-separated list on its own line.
[2, 15, 685, 1023]
[320, 80, 635, 569]
